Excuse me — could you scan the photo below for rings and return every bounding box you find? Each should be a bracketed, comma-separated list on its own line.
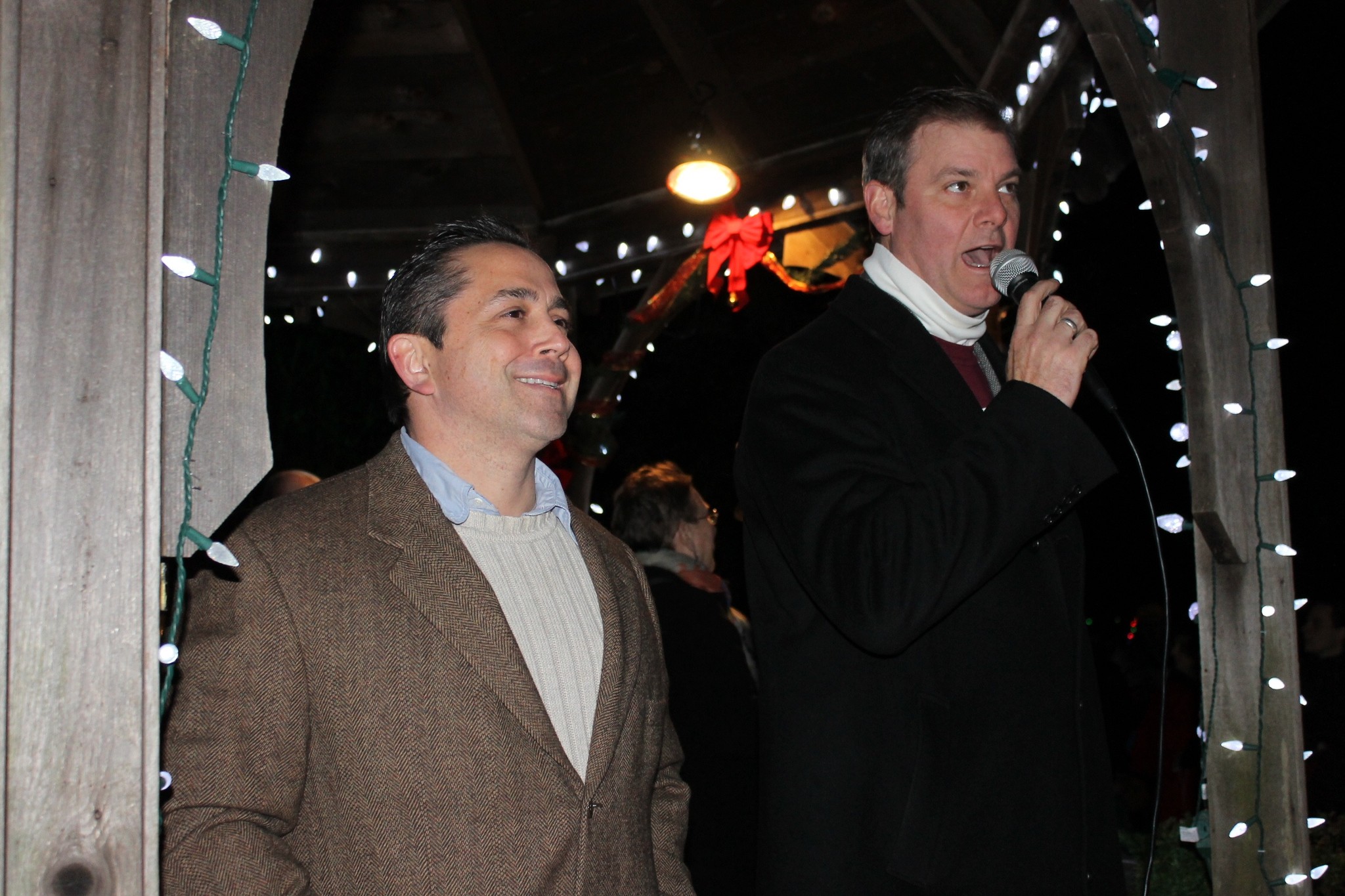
[1060, 318, 1077, 334]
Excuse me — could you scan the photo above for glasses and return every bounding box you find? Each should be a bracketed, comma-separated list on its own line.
[685, 507, 718, 525]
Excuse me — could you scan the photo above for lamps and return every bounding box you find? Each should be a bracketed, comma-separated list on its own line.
[664, 119, 743, 208]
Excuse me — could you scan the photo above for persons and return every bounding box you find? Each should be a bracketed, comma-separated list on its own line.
[1301, 604, 1345, 816]
[737, 97, 1117, 896]
[157, 212, 698, 896]
[256, 469, 321, 504]
[1128, 636, 1198, 818]
[614, 460, 760, 896]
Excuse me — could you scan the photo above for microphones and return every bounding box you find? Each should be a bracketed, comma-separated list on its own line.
[988, 248, 1120, 412]
